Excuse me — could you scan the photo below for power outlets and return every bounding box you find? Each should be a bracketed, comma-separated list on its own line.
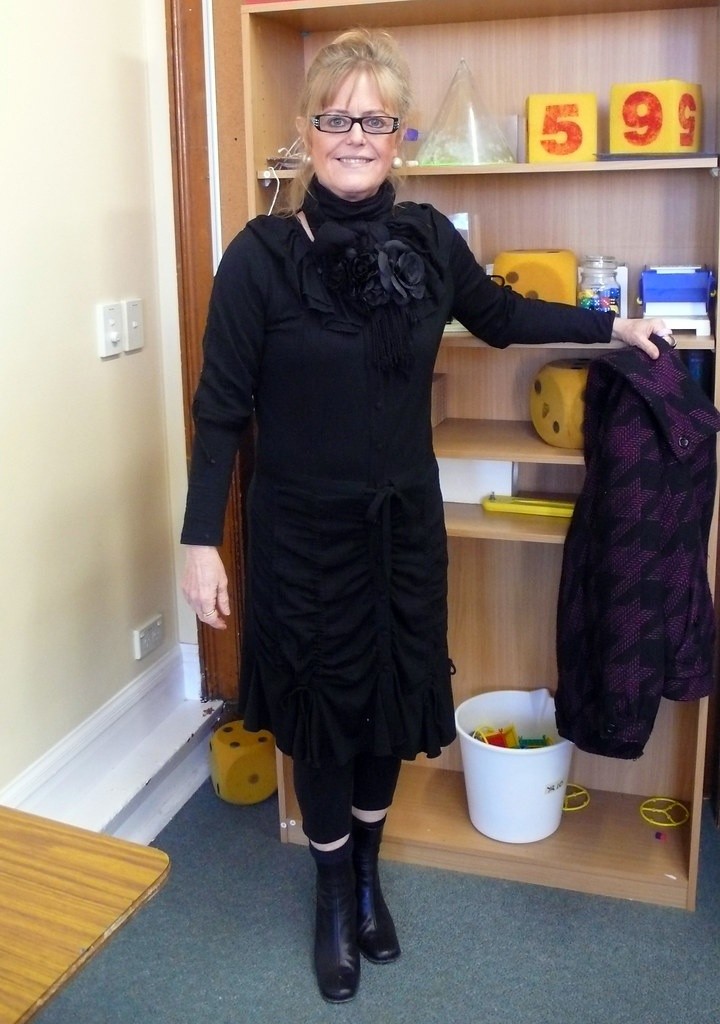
[134, 613, 165, 660]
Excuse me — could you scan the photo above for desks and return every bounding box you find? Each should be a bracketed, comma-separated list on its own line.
[0, 806, 171, 1024]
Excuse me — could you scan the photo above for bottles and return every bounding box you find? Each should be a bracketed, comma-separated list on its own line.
[577, 255, 621, 318]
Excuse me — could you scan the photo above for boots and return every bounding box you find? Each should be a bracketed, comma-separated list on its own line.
[348, 813, 401, 966]
[308, 834, 361, 1004]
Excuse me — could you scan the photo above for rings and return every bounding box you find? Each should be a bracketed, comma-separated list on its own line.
[204, 610, 215, 617]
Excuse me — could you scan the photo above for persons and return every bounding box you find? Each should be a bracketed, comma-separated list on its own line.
[178, 25, 677, 1006]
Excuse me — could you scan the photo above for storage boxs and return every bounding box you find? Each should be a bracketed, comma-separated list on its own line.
[455, 687, 574, 844]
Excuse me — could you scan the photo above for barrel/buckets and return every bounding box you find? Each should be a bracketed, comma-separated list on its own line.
[454, 689, 574, 844]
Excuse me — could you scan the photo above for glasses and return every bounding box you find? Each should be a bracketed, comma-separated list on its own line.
[308, 112, 401, 135]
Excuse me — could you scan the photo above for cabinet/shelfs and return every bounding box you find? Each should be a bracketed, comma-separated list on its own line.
[238, 3, 719, 913]
